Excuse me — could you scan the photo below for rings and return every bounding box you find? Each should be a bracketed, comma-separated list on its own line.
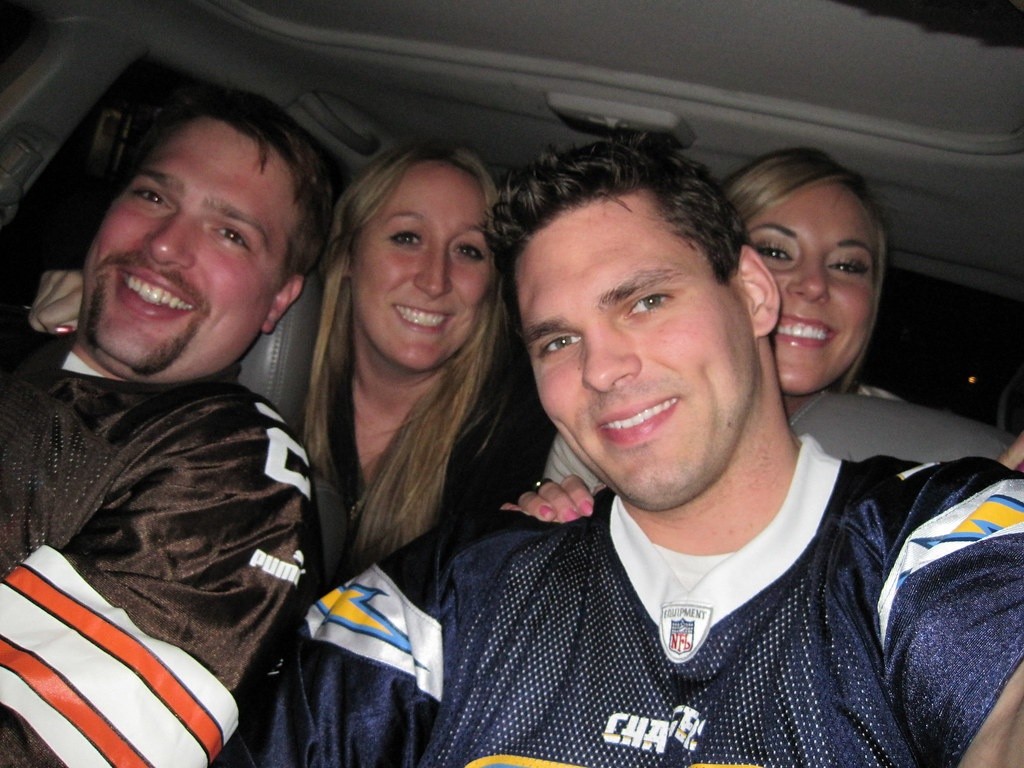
[535, 478, 551, 494]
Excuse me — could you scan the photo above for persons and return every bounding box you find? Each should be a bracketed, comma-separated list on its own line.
[492, 145, 886, 520]
[0, 91, 339, 768]
[216, 130, 1024, 768]
[26, 138, 552, 579]
